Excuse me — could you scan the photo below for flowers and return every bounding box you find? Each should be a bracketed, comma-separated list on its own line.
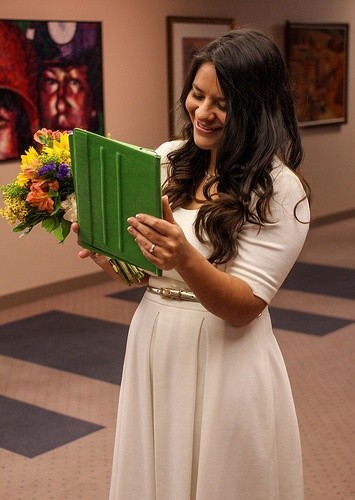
[4, 128, 146, 286]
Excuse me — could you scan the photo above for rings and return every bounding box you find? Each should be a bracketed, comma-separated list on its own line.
[148, 243, 155, 253]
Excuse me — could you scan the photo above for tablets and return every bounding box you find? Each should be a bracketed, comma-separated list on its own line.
[68, 128, 163, 277]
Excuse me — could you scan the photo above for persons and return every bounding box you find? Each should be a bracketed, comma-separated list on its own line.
[72, 28, 311, 500]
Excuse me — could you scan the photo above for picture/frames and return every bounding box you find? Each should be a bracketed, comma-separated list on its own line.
[284, 21, 349, 127]
[165, 14, 235, 143]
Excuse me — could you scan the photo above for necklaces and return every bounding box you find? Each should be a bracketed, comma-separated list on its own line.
[202, 170, 216, 186]
